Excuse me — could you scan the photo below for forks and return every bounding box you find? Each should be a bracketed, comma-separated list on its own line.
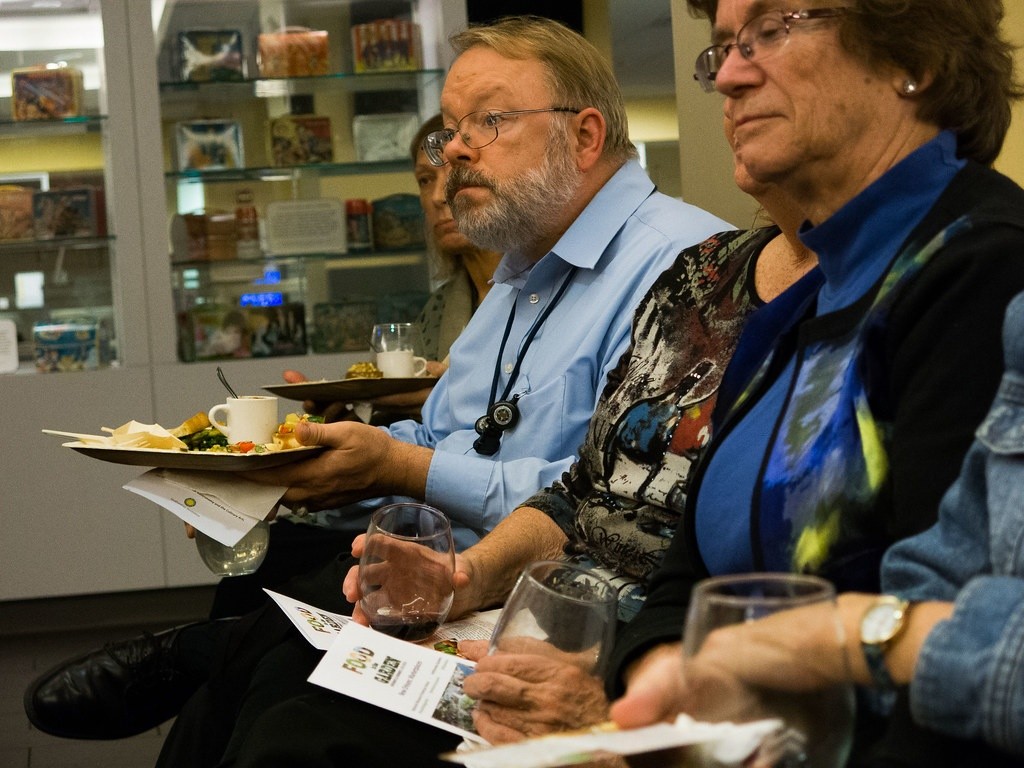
[42, 430, 151, 448]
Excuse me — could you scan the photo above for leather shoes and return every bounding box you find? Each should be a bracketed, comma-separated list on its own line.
[22, 624, 211, 742]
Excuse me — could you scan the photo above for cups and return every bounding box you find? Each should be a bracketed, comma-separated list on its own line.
[377, 351, 427, 377]
[683, 573, 854, 768]
[489, 561, 617, 683]
[370, 323, 428, 369]
[209, 396, 279, 446]
[358, 503, 455, 641]
[196, 516, 270, 577]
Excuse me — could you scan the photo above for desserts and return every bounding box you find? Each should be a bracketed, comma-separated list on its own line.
[346, 361, 384, 379]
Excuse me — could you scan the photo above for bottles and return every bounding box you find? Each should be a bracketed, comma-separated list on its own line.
[236, 188, 259, 259]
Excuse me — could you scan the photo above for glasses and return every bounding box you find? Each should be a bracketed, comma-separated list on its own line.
[692, 5, 856, 94]
[421, 105, 584, 168]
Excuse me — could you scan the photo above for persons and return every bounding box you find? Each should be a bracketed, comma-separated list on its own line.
[21, 0, 1023, 768]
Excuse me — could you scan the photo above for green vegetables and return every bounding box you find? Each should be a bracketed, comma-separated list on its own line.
[180, 428, 228, 452]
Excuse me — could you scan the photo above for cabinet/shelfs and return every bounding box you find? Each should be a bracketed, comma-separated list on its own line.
[0, 0, 167, 601]
[128, 0, 469, 589]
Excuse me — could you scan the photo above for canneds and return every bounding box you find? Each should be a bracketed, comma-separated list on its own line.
[169, 411, 210, 438]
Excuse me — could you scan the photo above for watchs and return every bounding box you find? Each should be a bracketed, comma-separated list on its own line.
[855, 598, 911, 719]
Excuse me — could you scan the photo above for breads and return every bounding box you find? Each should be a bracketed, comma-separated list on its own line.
[272, 432, 305, 450]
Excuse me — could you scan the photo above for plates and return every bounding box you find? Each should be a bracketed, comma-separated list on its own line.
[261, 377, 440, 402]
[61, 427, 328, 470]
